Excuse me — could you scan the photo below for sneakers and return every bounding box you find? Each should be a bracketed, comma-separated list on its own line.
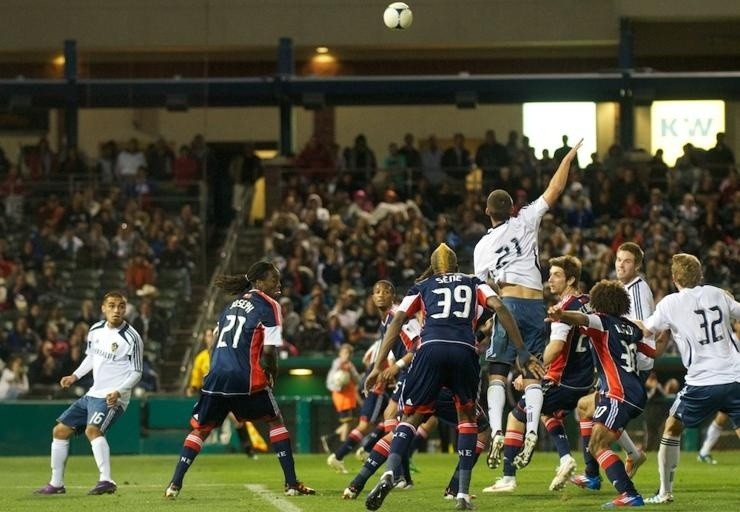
[481, 477, 519, 494]
[409, 458, 420, 474]
[548, 458, 602, 493]
[283, 481, 317, 497]
[164, 480, 182, 499]
[365, 473, 415, 512]
[356, 447, 371, 465]
[486, 429, 539, 470]
[342, 483, 362, 500]
[88, 480, 118, 496]
[603, 490, 675, 507]
[33, 483, 66, 495]
[624, 451, 647, 480]
[320, 435, 348, 475]
[443, 487, 477, 510]
[696, 453, 719, 465]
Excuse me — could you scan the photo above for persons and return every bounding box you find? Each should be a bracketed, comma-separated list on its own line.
[696, 408, 740, 464]
[1, 129, 740, 402]
[187, 329, 256, 459]
[36, 290, 144, 496]
[634, 253, 740, 504]
[321, 140, 672, 511]
[162, 259, 316, 497]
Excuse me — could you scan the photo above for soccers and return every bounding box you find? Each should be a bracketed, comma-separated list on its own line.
[383, 2, 413, 32]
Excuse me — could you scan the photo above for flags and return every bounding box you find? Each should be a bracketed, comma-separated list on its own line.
[245, 420, 272, 454]
